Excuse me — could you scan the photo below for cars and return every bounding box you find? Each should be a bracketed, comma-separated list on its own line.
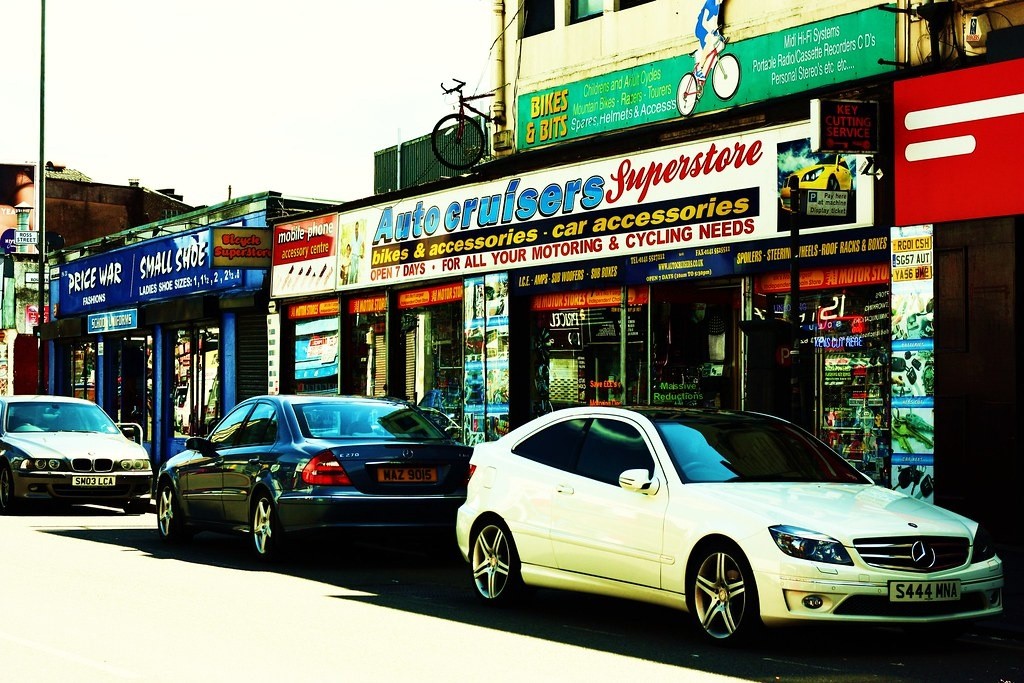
[174, 387, 186, 426]
[0, 393, 152, 511]
[453, 405, 1007, 649]
[779, 153, 852, 214]
[152, 391, 473, 565]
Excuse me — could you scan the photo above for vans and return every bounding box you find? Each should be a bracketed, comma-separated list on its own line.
[180, 368, 215, 435]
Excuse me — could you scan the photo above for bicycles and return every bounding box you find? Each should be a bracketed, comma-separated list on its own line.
[432, 78, 497, 171]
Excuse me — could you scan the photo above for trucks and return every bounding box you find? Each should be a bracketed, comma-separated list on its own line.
[205, 373, 220, 434]
